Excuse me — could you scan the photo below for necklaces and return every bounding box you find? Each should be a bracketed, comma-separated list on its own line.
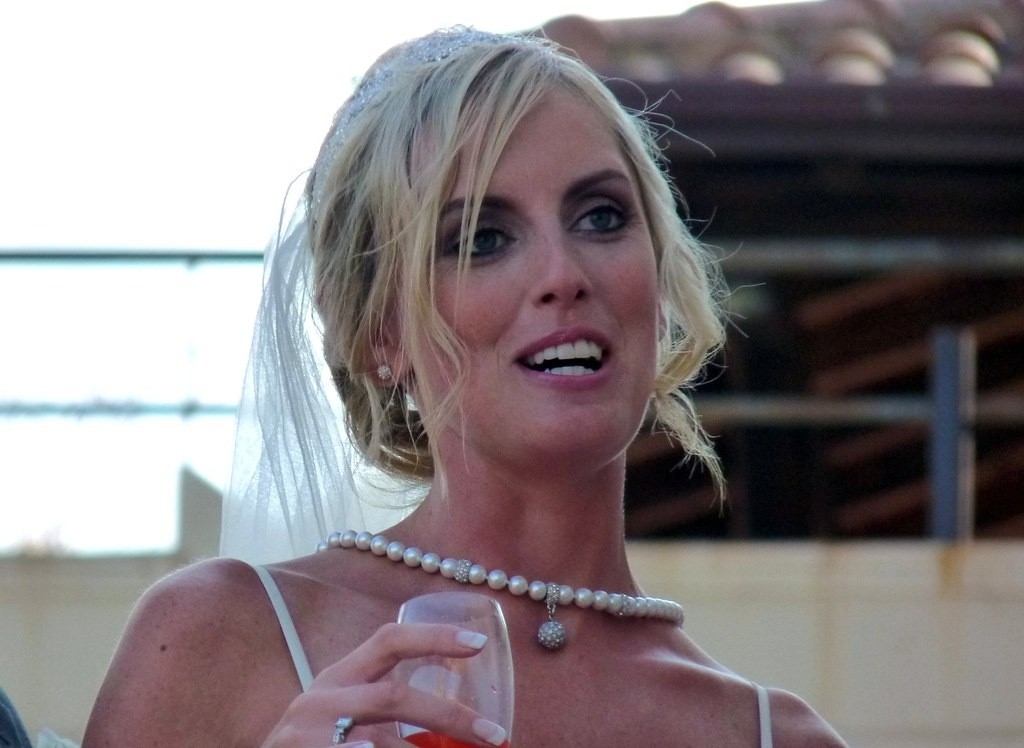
[318, 529, 683, 651]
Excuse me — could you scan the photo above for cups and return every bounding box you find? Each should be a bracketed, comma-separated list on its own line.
[392, 592, 515, 748]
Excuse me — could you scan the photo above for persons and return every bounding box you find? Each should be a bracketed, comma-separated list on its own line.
[82, 27, 853, 748]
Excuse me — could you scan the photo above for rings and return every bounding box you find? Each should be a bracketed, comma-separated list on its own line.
[333, 716, 355, 743]
